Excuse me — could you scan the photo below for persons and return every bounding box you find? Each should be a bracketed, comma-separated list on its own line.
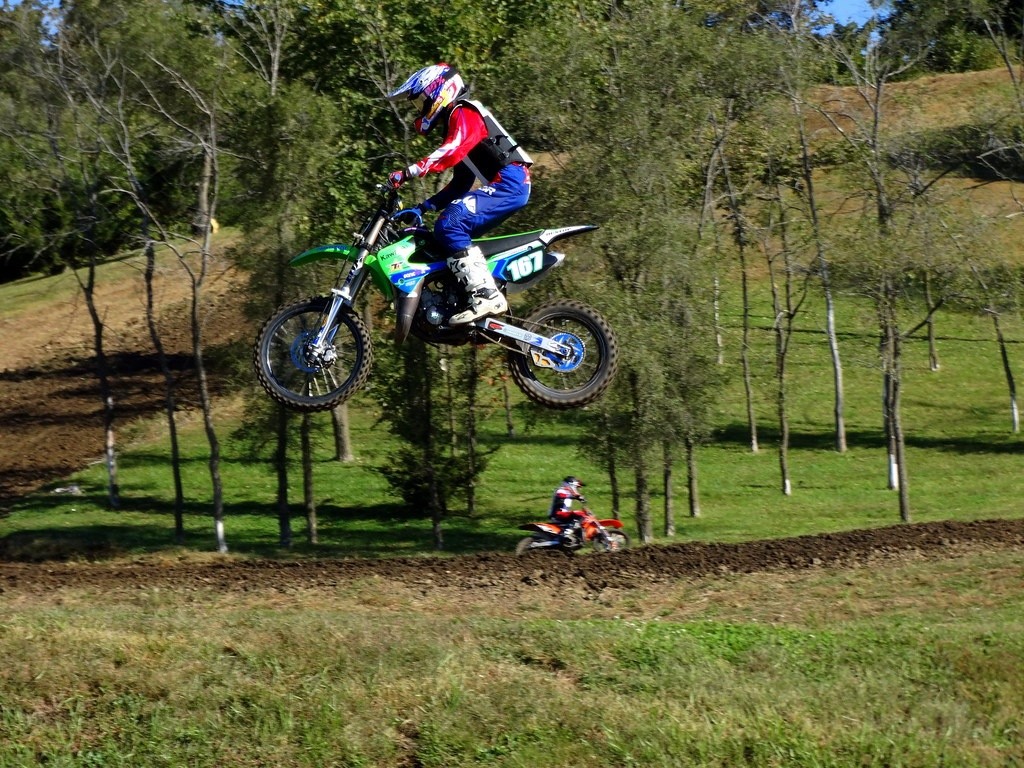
[549, 475, 586, 543]
[385, 62, 534, 326]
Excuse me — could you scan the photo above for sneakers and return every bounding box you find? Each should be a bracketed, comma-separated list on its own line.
[445, 242, 509, 328]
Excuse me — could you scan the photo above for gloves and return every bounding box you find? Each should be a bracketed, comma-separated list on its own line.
[385, 168, 413, 190]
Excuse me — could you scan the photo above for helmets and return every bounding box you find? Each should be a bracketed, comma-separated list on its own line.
[386, 61, 467, 137]
[562, 475, 587, 495]
